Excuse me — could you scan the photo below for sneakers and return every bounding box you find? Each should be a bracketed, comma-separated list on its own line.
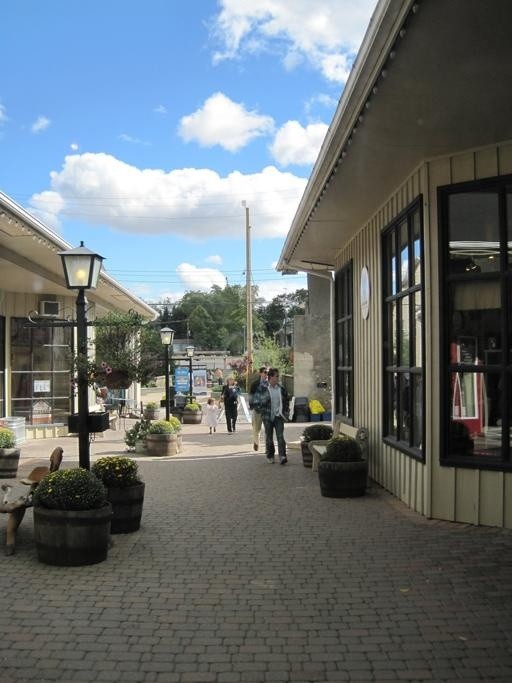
[265, 453, 274, 464]
[279, 454, 289, 465]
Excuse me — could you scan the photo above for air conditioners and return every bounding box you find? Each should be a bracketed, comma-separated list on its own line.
[38, 298, 62, 317]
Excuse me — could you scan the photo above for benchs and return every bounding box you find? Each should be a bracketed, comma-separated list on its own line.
[308, 418, 367, 474]
[1, 444, 63, 556]
[25, 422, 64, 438]
[95, 411, 120, 437]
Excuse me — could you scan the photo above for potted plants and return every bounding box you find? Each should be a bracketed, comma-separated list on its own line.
[317, 434, 369, 497]
[301, 422, 334, 467]
[0, 427, 20, 480]
[29, 466, 113, 566]
[89, 455, 145, 535]
[124, 400, 203, 455]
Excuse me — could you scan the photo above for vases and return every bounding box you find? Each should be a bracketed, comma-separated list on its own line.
[99, 368, 133, 390]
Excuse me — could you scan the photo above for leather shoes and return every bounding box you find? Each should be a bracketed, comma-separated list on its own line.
[253, 442, 258, 451]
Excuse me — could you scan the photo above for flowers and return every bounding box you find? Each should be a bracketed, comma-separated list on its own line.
[66, 306, 159, 388]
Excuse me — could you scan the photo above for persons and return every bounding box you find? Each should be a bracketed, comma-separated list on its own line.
[201, 367, 292, 465]
[97, 387, 110, 404]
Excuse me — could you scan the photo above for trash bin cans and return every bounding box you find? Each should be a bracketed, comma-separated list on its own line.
[294, 397, 309, 422]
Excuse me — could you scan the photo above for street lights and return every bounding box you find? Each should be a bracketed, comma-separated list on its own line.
[157, 325, 176, 424]
[56, 237, 109, 480]
[185, 344, 197, 402]
